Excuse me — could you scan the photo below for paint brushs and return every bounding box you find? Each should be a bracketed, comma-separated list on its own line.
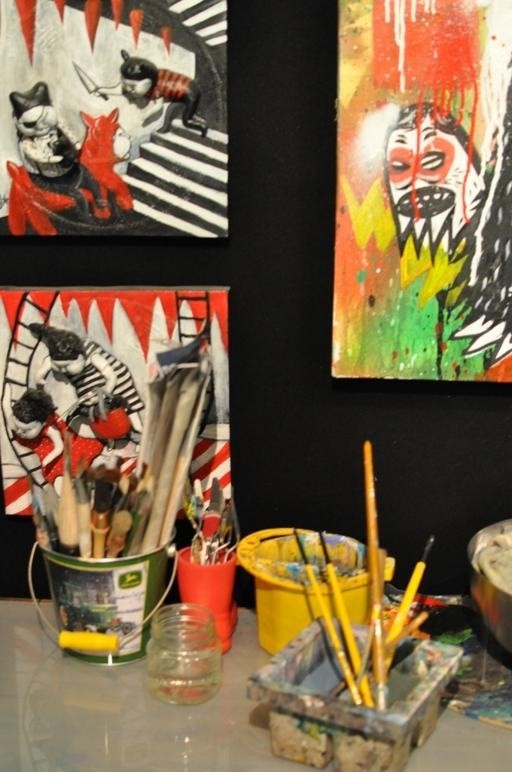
[27, 354, 231, 564]
[294, 429, 440, 713]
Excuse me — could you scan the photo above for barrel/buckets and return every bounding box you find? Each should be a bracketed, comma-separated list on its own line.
[235, 529, 384, 654]
[28, 533, 178, 666]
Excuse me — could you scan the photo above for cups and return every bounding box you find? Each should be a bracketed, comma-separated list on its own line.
[175, 546, 238, 655]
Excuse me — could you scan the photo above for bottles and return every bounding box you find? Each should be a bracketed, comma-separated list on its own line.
[146, 603, 224, 704]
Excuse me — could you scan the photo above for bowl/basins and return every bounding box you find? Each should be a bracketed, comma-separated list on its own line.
[465, 517, 512, 659]
